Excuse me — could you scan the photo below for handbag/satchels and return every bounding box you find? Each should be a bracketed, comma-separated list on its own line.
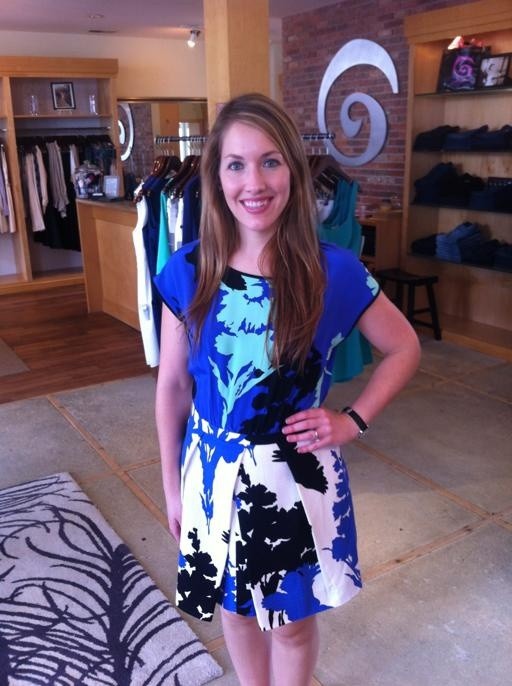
[435, 46, 492, 93]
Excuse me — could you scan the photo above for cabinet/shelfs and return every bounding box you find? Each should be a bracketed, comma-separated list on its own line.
[398, 0, 512, 362]
[1, 55, 126, 313]
[358, 214, 401, 300]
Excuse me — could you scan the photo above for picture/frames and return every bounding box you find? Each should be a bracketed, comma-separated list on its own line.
[50, 81, 76, 110]
[476, 52, 511, 89]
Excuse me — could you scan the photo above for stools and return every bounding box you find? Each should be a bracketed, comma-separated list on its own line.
[372, 268, 442, 341]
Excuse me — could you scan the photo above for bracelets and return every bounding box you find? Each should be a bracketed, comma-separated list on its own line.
[342, 403, 370, 438]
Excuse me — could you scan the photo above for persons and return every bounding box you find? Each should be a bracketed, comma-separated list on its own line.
[151, 95, 426, 685]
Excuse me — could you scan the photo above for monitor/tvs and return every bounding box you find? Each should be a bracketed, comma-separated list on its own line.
[103, 175, 119, 198]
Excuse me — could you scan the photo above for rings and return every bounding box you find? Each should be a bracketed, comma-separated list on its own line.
[313, 428, 320, 440]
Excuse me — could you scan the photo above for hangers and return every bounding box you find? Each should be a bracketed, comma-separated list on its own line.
[129, 131, 362, 205]
[16, 126, 114, 153]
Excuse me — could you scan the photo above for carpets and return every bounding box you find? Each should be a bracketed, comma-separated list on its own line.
[0, 468, 224, 684]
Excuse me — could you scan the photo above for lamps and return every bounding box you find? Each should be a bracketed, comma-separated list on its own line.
[187, 29, 200, 49]
